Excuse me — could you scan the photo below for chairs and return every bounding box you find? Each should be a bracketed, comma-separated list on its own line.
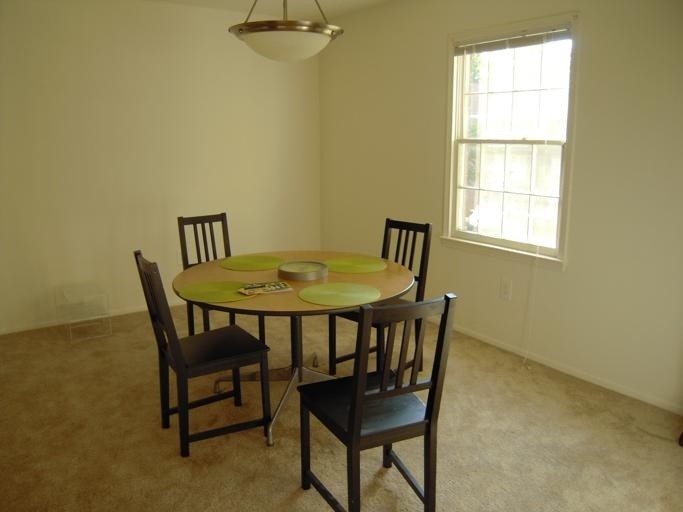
[178, 213, 235, 332]
[132, 249, 276, 454]
[326, 217, 432, 380]
[296, 290, 459, 512]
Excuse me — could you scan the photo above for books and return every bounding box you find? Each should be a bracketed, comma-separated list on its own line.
[236, 280, 295, 298]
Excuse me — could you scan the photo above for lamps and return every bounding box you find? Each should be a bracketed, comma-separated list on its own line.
[228, 1, 343, 65]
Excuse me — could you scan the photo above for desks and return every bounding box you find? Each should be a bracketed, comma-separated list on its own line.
[171, 250, 417, 410]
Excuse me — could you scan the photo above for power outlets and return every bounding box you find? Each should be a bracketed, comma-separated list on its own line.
[499, 278, 513, 301]
